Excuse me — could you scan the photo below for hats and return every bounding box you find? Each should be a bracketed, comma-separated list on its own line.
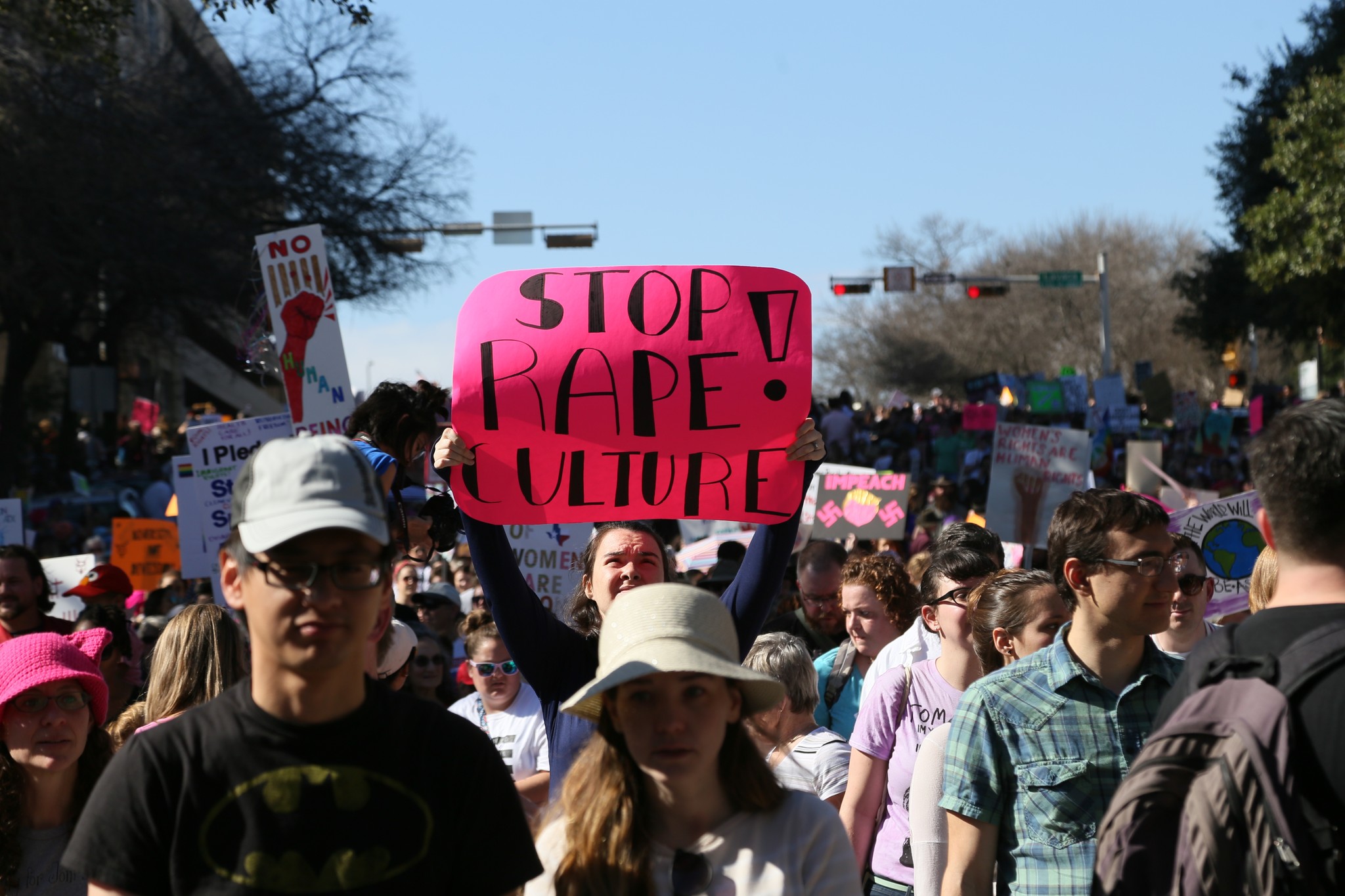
[410, 582, 461, 607]
[0, 628, 113, 728]
[230, 432, 390, 554]
[558, 582, 784, 725]
[393, 560, 416, 583]
[61, 563, 133, 599]
[377, 616, 418, 679]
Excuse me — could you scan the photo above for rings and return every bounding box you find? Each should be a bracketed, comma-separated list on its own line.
[811, 441, 818, 452]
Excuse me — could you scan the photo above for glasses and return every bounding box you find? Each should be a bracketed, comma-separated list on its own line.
[672, 848, 713, 896]
[419, 600, 451, 611]
[1083, 553, 1190, 577]
[248, 558, 389, 590]
[8, 692, 92, 713]
[411, 654, 445, 668]
[469, 659, 519, 677]
[802, 590, 839, 607]
[414, 548, 427, 554]
[399, 576, 419, 583]
[926, 587, 973, 607]
[1174, 574, 1208, 596]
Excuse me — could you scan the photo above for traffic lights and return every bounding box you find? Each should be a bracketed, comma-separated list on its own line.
[965, 284, 1008, 299]
[834, 283, 870, 295]
[1229, 370, 1247, 389]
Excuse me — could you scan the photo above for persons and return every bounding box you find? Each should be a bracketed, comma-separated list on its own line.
[0, 380, 1345, 896]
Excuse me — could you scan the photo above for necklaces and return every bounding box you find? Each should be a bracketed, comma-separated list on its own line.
[768, 724, 812, 766]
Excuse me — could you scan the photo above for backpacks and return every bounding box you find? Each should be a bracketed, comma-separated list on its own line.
[1090, 621, 1345, 896]
